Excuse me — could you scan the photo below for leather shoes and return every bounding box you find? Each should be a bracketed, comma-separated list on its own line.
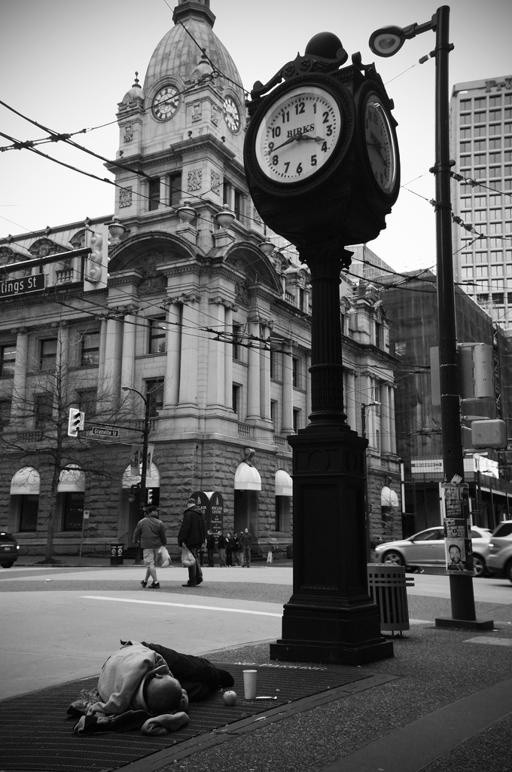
[182, 577, 204, 588]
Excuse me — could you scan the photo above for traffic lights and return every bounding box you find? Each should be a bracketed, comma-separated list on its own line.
[69, 408, 80, 436]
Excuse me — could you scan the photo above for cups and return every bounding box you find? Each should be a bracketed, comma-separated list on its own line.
[242, 668, 258, 700]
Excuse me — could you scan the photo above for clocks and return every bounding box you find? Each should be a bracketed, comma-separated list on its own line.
[251, 79, 345, 184]
[222, 96, 241, 134]
[152, 85, 181, 122]
[360, 90, 399, 199]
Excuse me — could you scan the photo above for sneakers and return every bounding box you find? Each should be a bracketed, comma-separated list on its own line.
[200, 563, 251, 567]
[149, 581, 159, 589]
[139, 581, 147, 587]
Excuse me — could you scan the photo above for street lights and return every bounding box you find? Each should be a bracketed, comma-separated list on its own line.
[474, 467, 491, 524]
[366, 4, 496, 633]
[360, 401, 382, 436]
[122, 384, 151, 565]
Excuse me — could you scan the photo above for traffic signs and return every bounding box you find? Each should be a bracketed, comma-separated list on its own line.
[92, 428, 119, 437]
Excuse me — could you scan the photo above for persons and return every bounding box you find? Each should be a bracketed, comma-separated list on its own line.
[447, 544, 466, 571]
[132, 507, 167, 589]
[205, 528, 253, 566]
[98, 643, 235, 737]
[178, 497, 208, 589]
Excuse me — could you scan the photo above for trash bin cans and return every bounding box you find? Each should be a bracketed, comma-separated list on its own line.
[110, 543, 126, 565]
[367, 562, 415, 639]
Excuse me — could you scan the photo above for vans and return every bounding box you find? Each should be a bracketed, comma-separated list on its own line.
[0, 533, 20, 568]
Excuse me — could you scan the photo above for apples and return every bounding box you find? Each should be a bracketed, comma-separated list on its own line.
[220, 690, 239, 707]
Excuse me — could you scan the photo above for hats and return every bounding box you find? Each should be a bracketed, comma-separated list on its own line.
[149, 507, 160, 511]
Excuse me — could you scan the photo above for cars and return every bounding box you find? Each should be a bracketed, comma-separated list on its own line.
[374, 525, 494, 577]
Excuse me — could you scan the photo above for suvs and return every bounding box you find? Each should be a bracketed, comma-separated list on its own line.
[489, 519, 512, 584]
